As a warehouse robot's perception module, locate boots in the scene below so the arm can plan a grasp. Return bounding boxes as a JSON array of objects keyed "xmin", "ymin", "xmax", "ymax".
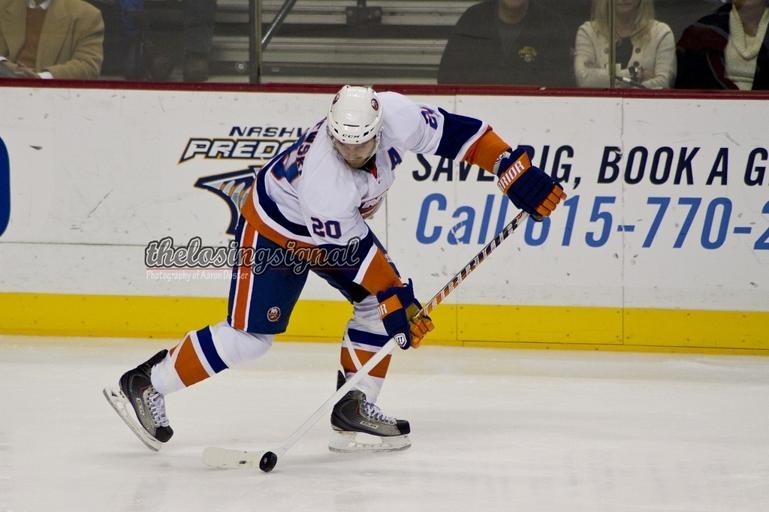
[
  {"xmin": 330, "ymin": 370, "xmax": 411, "ymax": 438},
  {"xmin": 119, "ymin": 348, "xmax": 173, "ymax": 442}
]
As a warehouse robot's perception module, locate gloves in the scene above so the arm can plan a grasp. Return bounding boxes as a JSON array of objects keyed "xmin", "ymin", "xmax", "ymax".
[
  {"xmin": 497, "ymin": 144, "xmax": 567, "ymax": 221},
  {"xmin": 376, "ymin": 278, "xmax": 434, "ymax": 351}
]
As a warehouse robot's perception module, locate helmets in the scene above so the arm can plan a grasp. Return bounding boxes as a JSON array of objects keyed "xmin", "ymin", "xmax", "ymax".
[{"xmin": 326, "ymin": 85, "xmax": 384, "ymax": 167}]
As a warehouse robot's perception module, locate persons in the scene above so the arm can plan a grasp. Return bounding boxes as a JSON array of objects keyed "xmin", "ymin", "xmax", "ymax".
[
  {"xmin": 119, "ymin": 83, "xmax": 570, "ymax": 439},
  {"xmin": 570, "ymin": 0, "xmax": 677, "ymax": 93},
  {"xmin": 437, "ymin": 1, "xmax": 576, "ymax": 90},
  {"xmin": 87, "ymin": 1, "xmax": 219, "ymax": 84},
  {"xmin": 674, "ymin": 0, "xmax": 768, "ymax": 92},
  {"xmin": 0, "ymin": 0, "xmax": 105, "ymax": 83}
]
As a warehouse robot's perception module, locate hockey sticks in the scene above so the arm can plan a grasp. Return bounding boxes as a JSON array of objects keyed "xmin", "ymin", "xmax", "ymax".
[{"xmin": 200, "ymin": 174, "xmax": 571, "ymax": 472}]
